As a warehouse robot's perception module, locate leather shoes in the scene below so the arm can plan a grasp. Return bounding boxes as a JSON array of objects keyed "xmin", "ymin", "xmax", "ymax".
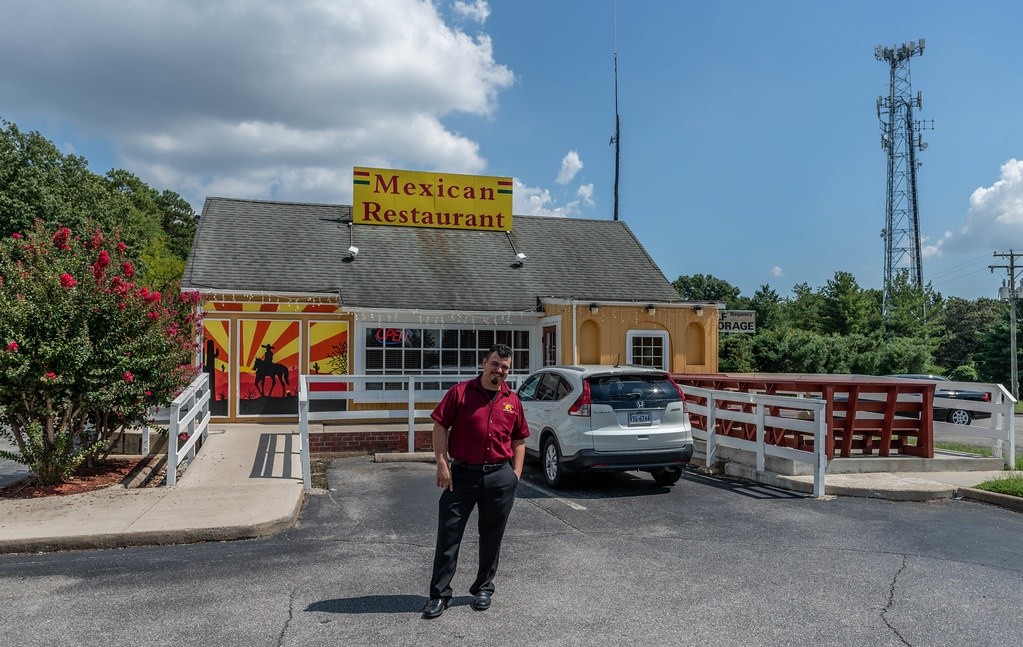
[
  {"xmin": 474, "ymin": 592, "xmax": 491, "ymax": 608},
  {"xmin": 424, "ymin": 597, "xmax": 452, "ymax": 616}
]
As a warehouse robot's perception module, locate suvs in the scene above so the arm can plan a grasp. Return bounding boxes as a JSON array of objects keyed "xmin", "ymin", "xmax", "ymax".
[{"xmin": 514, "ymin": 364, "xmax": 694, "ymax": 486}]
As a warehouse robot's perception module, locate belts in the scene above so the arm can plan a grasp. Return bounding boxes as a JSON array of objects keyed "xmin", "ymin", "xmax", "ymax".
[{"xmin": 452, "ymin": 458, "xmax": 506, "ymax": 472}]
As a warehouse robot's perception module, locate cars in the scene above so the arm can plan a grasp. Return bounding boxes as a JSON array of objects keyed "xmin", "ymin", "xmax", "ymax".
[{"xmin": 813, "ymin": 374, "xmax": 992, "ymax": 425}]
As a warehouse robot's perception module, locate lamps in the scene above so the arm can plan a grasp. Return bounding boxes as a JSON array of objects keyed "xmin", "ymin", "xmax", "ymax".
[
  {"xmin": 348, "ymin": 246, "xmax": 359, "ymax": 258},
  {"xmin": 589, "ymin": 304, "xmax": 598, "ymax": 315},
  {"xmin": 646, "ymin": 306, "xmax": 655, "ymax": 315},
  {"xmin": 515, "ymin": 252, "xmax": 527, "ymax": 266},
  {"xmin": 694, "ymin": 307, "xmax": 703, "ymax": 316}
]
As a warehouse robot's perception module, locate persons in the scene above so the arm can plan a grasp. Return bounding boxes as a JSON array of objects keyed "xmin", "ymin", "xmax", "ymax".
[{"xmin": 424, "ymin": 344, "xmax": 531, "ymax": 619}]
